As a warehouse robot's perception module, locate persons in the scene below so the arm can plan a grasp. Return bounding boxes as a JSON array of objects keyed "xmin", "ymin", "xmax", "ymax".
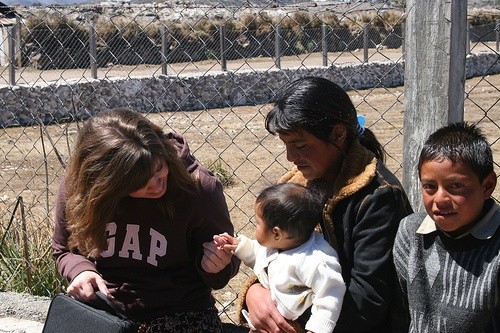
[
  {"xmin": 213, "ymin": 182, "xmax": 348, "ymax": 333},
  {"xmin": 234, "ymin": 76, "xmax": 416, "ymax": 333},
  {"xmin": 49, "ymin": 107, "xmax": 243, "ymax": 333},
  {"xmin": 391, "ymin": 122, "xmax": 500, "ymax": 333}
]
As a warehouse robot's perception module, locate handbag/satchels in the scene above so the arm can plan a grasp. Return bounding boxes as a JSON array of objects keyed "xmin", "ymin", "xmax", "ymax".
[{"xmin": 42, "ymin": 289, "xmax": 139, "ymax": 333}]
